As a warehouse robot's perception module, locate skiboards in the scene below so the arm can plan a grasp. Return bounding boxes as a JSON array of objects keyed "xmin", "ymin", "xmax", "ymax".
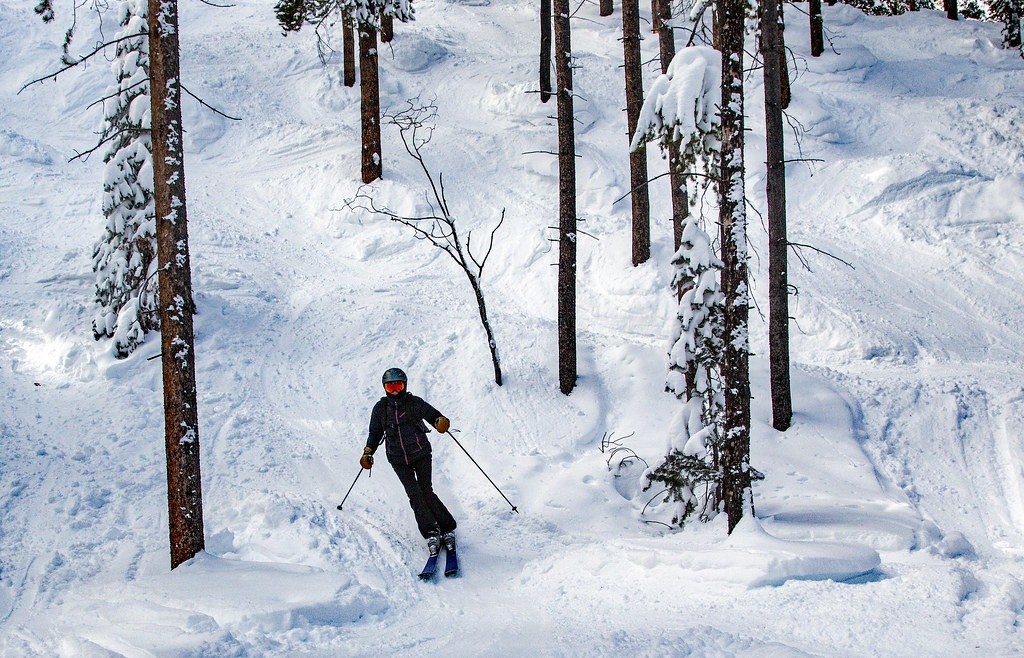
[{"xmin": 417, "ymin": 529, "xmax": 459, "ymax": 580}]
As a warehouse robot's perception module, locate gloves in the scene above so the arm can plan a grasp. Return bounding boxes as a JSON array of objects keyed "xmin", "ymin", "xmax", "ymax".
[
  {"xmin": 435, "ymin": 416, "xmax": 449, "ymax": 433},
  {"xmin": 360, "ymin": 447, "xmax": 373, "ymax": 469}
]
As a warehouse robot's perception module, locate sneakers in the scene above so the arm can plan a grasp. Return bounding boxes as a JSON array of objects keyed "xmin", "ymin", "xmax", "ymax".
[
  {"xmin": 443, "ymin": 531, "xmax": 455, "ymax": 551},
  {"xmin": 428, "ymin": 535, "xmax": 441, "ymax": 554}
]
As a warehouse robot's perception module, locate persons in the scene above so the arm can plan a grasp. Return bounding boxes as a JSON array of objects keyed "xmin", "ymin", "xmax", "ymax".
[{"xmin": 360, "ymin": 368, "xmax": 457, "ymax": 557}]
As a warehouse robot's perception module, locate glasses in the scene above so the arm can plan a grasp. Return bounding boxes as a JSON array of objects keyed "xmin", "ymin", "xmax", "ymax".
[{"xmin": 385, "ymin": 381, "xmax": 404, "ymax": 393}]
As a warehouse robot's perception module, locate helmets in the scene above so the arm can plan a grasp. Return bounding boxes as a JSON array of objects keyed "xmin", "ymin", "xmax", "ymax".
[{"xmin": 382, "ymin": 368, "xmax": 407, "ymax": 385}]
